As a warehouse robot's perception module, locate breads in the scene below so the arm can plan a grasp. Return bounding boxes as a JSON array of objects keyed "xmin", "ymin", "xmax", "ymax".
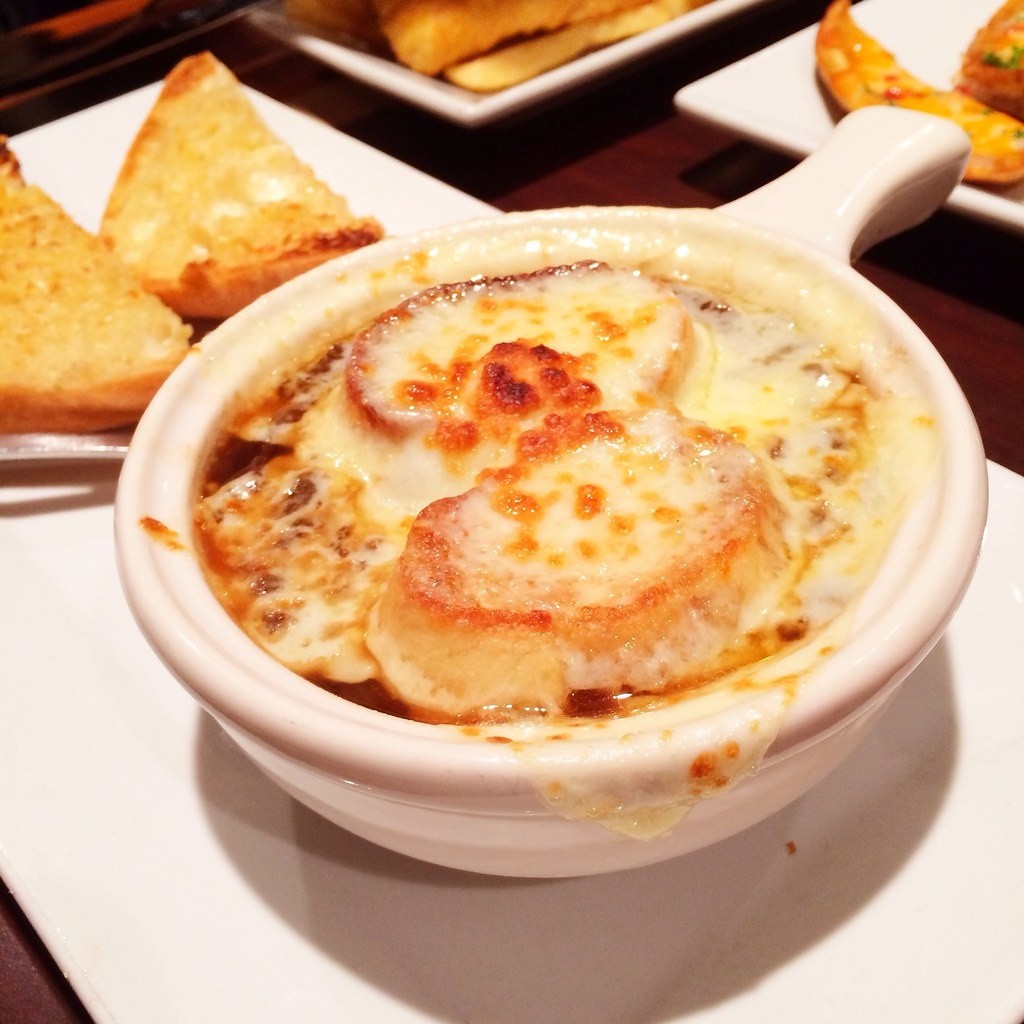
[
  {"xmin": 0, "ymin": 135, "xmax": 195, "ymax": 435},
  {"xmin": 271, "ymin": 1, "xmax": 710, "ymax": 97},
  {"xmin": 92, "ymin": 49, "xmax": 382, "ymax": 324}
]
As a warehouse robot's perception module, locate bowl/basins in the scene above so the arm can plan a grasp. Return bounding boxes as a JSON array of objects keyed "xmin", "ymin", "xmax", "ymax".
[{"xmin": 115, "ymin": 106, "xmax": 990, "ymax": 877}]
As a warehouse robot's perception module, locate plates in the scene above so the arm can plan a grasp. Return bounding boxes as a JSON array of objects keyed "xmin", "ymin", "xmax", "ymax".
[
  {"xmin": 675, "ymin": 0, "xmax": 1024, "ymax": 234},
  {"xmin": 0, "ymin": 72, "xmax": 1024, "ymax": 1024},
  {"xmin": 242, "ymin": 1, "xmax": 764, "ymax": 125}
]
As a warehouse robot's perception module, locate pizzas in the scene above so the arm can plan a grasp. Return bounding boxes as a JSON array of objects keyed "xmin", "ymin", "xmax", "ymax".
[
  {"xmin": 954, "ymin": 0, "xmax": 1024, "ymax": 126},
  {"xmin": 814, "ymin": 0, "xmax": 1024, "ymax": 188}
]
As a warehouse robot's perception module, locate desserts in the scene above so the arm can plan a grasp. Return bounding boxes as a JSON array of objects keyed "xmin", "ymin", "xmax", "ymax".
[{"xmin": 192, "ymin": 248, "xmax": 904, "ymax": 731}]
